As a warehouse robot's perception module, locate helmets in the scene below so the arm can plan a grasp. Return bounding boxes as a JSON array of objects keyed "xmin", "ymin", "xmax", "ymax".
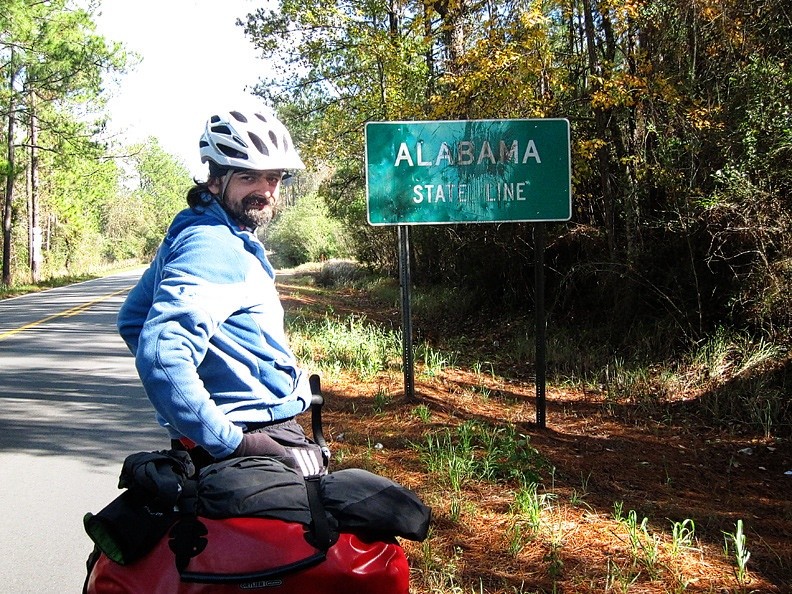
[{"xmin": 198, "ymin": 109, "xmax": 307, "ymax": 171}]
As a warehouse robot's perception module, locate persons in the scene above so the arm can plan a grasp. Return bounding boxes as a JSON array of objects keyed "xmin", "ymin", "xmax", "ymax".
[{"xmin": 116, "ymin": 108, "xmax": 330, "ymax": 477}]
